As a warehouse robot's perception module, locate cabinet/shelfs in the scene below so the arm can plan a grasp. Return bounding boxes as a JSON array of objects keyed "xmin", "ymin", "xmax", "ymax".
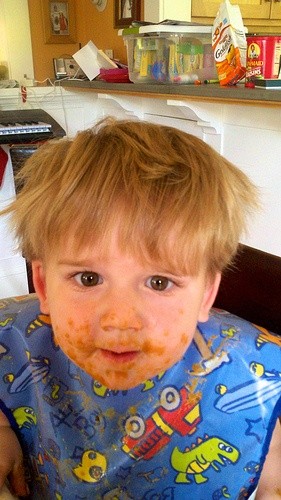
[
  {"xmin": 0, "ymin": 78, "xmax": 281, "ymax": 258},
  {"xmin": 192, "ymin": 0, "xmax": 281, "ymax": 34}
]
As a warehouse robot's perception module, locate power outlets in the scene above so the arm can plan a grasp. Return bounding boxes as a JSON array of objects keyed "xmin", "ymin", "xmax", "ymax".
[{"xmin": 105, "ymin": 50, "xmax": 113, "ymax": 58}]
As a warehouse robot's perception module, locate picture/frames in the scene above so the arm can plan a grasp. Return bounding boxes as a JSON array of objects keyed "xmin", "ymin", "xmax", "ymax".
[
  {"xmin": 40, "ymin": 0, "xmax": 77, "ymax": 44},
  {"xmin": 114, "ymin": 0, "xmax": 144, "ymax": 30}
]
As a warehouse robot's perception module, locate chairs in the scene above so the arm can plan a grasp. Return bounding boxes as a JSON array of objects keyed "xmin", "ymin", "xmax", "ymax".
[{"xmin": 212, "ymin": 243, "xmax": 281, "ymax": 336}]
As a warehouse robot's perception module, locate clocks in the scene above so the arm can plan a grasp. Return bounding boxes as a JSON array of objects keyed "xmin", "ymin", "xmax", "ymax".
[{"xmin": 94, "ymin": 0, "xmax": 107, "ymax": 12}]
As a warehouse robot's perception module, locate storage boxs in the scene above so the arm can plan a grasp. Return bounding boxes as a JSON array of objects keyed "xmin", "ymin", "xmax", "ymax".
[{"xmin": 117, "ymin": 24, "xmax": 248, "ymax": 85}]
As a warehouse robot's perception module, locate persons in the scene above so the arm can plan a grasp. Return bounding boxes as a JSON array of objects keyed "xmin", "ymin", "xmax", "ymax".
[
  {"xmin": 58, "ymin": 11, "xmax": 68, "ymax": 34},
  {"xmin": 0, "ymin": 117, "xmax": 280, "ymax": 500}
]
